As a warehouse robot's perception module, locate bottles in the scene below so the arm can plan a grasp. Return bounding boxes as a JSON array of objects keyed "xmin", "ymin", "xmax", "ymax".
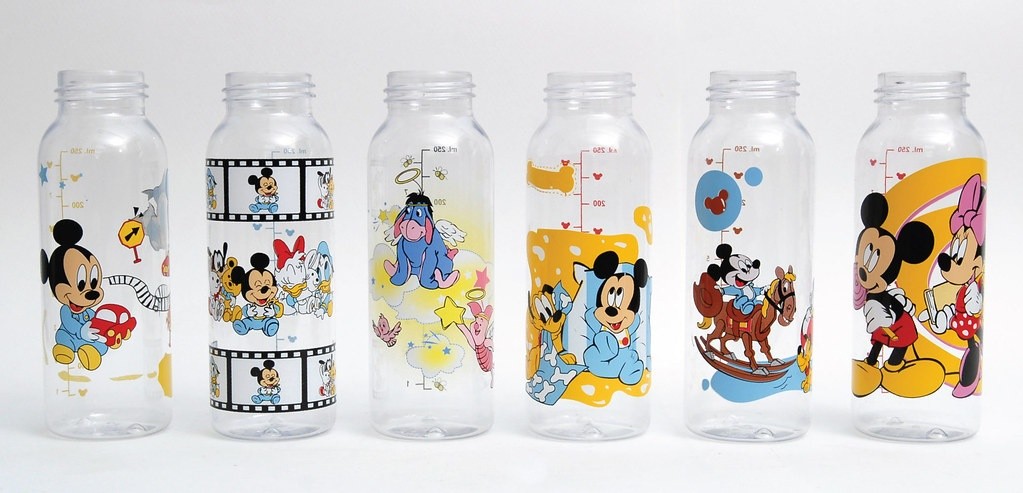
[
  {"xmin": 37, "ymin": 66, "xmax": 171, "ymax": 443},
  {"xmin": 527, "ymin": 70, "xmax": 655, "ymax": 442},
  {"xmin": 203, "ymin": 68, "xmax": 337, "ymax": 439},
  {"xmin": 684, "ymin": 67, "xmax": 816, "ymax": 444},
  {"xmin": 847, "ymin": 68, "xmax": 988, "ymax": 442},
  {"xmin": 364, "ymin": 68, "xmax": 493, "ymax": 445}
]
